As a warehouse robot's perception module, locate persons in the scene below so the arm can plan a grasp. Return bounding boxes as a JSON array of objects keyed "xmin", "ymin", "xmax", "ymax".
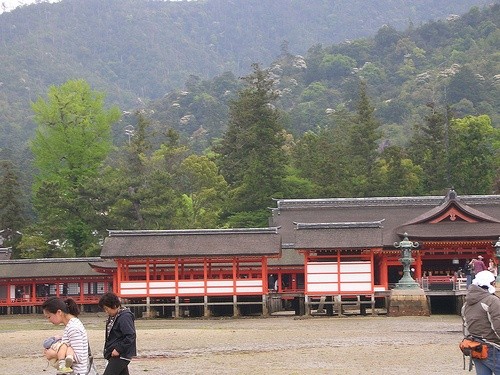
[
  {"xmin": 465, "ymin": 256, "xmax": 498, "ymax": 288},
  {"xmin": 43, "ymin": 337, "xmax": 74, "ymax": 372},
  {"xmin": 459, "ymin": 270, "xmax": 500, "ymax": 375},
  {"xmin": 42, "ymin": 297, "xmax": 93, "ymax": 375},
  {"xmin": 99, "ymin": 293, "xmax": 136, "ymax": 375},
  {"xmin": 268, "ymin": 274, "xmax": 285, "ymax": 291}
]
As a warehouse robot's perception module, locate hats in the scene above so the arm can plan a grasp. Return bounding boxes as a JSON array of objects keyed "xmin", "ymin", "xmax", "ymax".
[
  {"xmin": 471, "ymin": 270, "xmax": 495, "ymax": 294},
  {"xmin": 478, "ymin": 256, "xmax": 483, "ymax": 259}
]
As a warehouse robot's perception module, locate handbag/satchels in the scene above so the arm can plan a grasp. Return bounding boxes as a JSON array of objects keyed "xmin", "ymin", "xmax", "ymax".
[{"xmin": 458, "ymin": 334, "xmax": 488, "ymax": 360}]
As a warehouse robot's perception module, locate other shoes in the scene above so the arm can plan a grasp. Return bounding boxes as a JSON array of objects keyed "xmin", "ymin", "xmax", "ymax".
[{"xmin": 58, "ymin": 355, "xmax": 74, "ymax": 373}]
